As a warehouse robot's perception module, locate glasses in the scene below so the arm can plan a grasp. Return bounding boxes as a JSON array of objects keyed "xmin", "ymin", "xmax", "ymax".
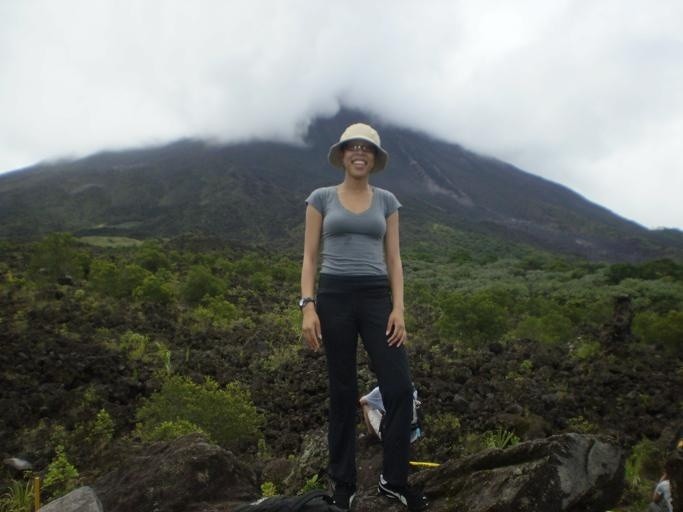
[{"xmin": 343, "ymin": 144, "xmax": 377, "ymax": 154}]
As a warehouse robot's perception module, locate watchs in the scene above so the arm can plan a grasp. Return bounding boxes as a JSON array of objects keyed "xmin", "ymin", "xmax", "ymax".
[{"xmin": 298, "ymin": 297, "xmax": 316, "ymax": 310}]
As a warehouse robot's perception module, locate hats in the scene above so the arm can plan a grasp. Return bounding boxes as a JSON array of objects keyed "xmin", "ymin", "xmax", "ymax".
[{"xmin": 327, "ymin": 123, "xmax": 389, "ymax": 172}]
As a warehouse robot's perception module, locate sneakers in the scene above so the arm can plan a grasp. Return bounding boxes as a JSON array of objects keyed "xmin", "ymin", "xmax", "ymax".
[
  {"xmin": 329, "ymin": 479, "xmax": 359, "ymax": 511},
  {"xmin": 376, "ymin": 473, "xmax": 429, "ymax": 512}
]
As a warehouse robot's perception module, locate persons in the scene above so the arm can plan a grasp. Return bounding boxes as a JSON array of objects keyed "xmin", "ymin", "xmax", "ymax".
[
  {"xmin": 301, "ymin": 122, "xmax": 430, "ymax": 512},
  {"xmin": 648, "ymin": 457, "xmax": 683, "ymax": 512},
  {"xmin": 358, "ymin": 381, "xmax": 422, "ymax": 445}
]
